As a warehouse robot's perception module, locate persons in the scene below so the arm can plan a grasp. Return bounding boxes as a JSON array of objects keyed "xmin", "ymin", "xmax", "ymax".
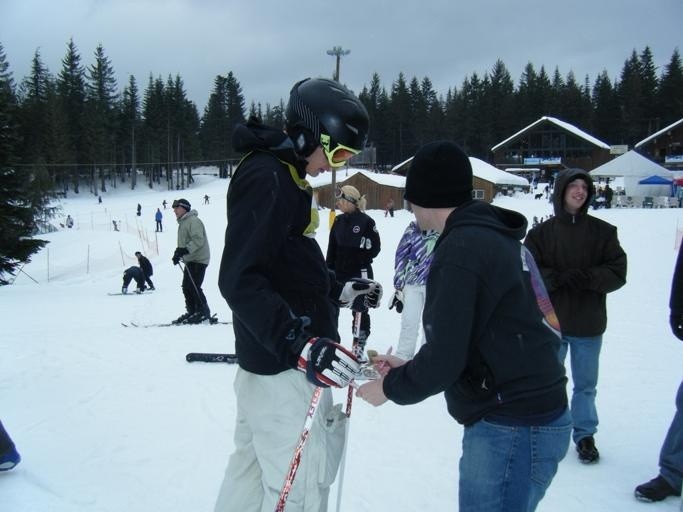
[
  {"xmin": 134, "ymin": 251, "xmax": 155, "ymax": 293},
  {"xmin": 351, "ymin": 138, "xmax": 579, "ymax": 511},
  {"xmin": 543, "ymin": 183, "xmax": 549, "ymax": 200},
  {"xmin": 65, "ymin": 215, "xmax": 72, "ymax": 228},
  {"xmin": 161, "ymin": 199, "xmax": 168, "ymax": 209},
  {"xmin": 212, "ymin": 74, "xmax": 383, "ymax": 512},
  {"xmin": 154, "ymin": 207, "xmax": 163, "ymax": 233},
  {"xmin": 534, "ymin": 191, "xmax": 542, "ymax": 199},
  {"xmin": 135, "ymin": 203, "xmax": 142, "ymax": 216},
  {"xmin": 603, "ymin": 184, "xmax": 613, "ymax": 208},
  {"xmin": 386, "ymin": 196, "xmax": 394, "ymax": 218},
  {"xmin": 525, "ymin": 168, "xmax": 629, "ymax": 466},
  {"xmin": 120, "ymin": 266, "xmax": 144, "ymax": 295},
  {"xmin": 170, "ymin": 198, "xmax": 211, "ymax": 325},
  {"xmin": 632, "ymin": 234, "xmax": 682, "ymax": 503},
  {"xmin": 203, "ymin": 195, "xmax": 210, "ymax": 204},
  {"xmin": 110, "ymin": 219, "xmax": 120, "ymax": 231},
  {"xmin": 386, "ymin": 218, "xmax": 439, "ymax": 364},
  {"xmin": 326, "ymin": 185, "xmax": 380, "ymax": 364},
  {"xmin": 0, "ymin": 419, "xmax": 20, "ymax": 472}
]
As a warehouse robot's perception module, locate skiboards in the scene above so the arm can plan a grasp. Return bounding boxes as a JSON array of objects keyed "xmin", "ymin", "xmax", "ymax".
[
  {"xmin": 109, "ymin": 292, "xmax": 154, "ymax": 296},
  {"xmin": 120, "ymin": 322, "xmax": 235, "ymax": 328}
]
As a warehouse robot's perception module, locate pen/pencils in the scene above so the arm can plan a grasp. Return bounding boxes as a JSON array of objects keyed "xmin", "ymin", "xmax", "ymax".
[{"xmin": 380, "ymin": 345, "xmax": 393, "ymax": 369}]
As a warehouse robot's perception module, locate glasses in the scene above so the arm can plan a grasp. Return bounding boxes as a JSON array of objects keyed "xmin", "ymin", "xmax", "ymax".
[
  {"xmin": 290, "ymin": 77, "xmax": 362, "ymax": 168},
  {"xmin": 172, "ymin": 200, "xmax": 190, "ymax": 208},
  {"xmin": 335, "ymin": 189, "xmax": 357, "ymax": 204}
]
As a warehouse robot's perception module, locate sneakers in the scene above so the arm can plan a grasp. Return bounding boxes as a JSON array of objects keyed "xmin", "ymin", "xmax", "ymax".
[
  {"xmin": 636, "ymin": 476, "xmax": 680, "ymax": 501},
  {"xmin": 577, "ymin": 436, "xmax": 599, "ymax": 462},
  {"xmin": 178, "ymin": 313, "xmax": 210, "ymax": 323}
]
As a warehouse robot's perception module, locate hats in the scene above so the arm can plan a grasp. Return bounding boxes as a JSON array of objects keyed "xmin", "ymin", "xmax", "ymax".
[{"xmin": 402, "ymin": 141, "xmax": 472, "ymax": 208}]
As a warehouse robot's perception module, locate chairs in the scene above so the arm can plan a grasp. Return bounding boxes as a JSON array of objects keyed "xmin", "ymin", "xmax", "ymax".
[{"xmin": 612, "ymin": 195, "xmax": 679, "ymax": 210}]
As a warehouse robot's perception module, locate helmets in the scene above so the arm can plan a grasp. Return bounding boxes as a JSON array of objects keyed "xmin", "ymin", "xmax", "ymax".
[
  {"xmin": 286, "ymin": 79, "xmax": 370, "ymax": 157},
  {"xmin": 178, "ymin": 199, "xmax": 190, "ymax": 211}
]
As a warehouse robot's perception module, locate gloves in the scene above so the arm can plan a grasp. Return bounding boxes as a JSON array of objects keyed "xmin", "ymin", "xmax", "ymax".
[
  {"xmin": 339, "ymin": 277, "xmax": 382, "ymax": 312},
  {"xmin": 172, "ymin": 248, "xmax": 188, "ymax": 265},
  {"xmin": 297, "ymin": 336, "xmax": 359, "ymax": 388},
  {"xmin": 388, "ymin": 289, "xmax": 404, "ymax": 313},
  {"xmin": 557, "ymin": 269, "xmax": 592, "ymax": 292}
]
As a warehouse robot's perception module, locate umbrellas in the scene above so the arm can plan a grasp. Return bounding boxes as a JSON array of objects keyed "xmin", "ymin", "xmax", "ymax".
[{"xmin": 637, "ymin": 173, "xmax": 673, "ymax": 207}]
